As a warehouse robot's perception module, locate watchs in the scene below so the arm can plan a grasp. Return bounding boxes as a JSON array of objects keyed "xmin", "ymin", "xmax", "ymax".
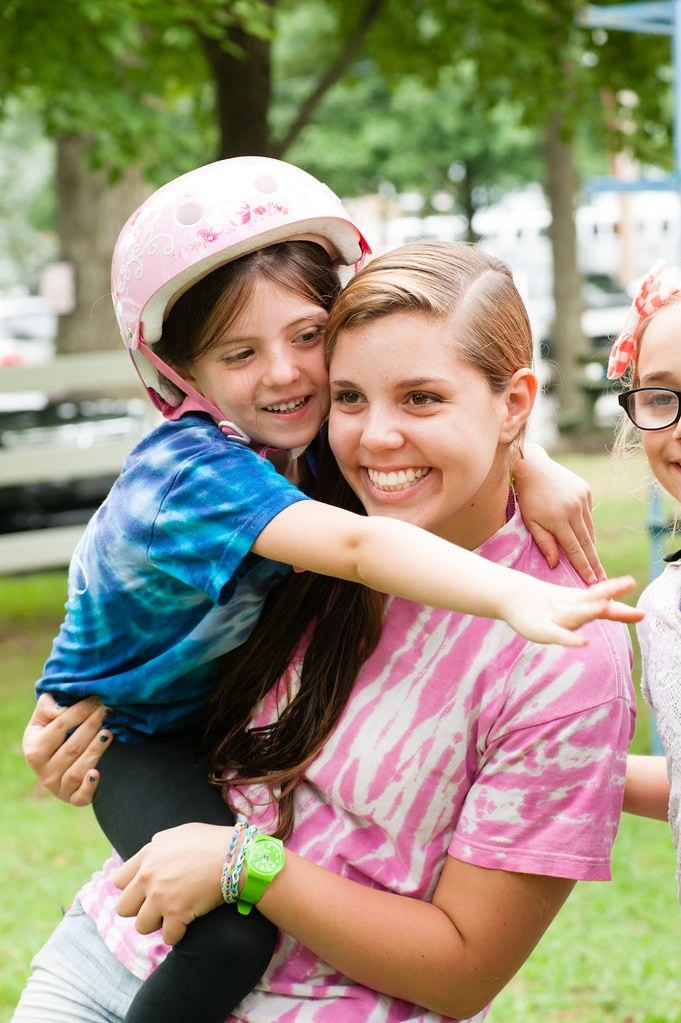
[{"xmin": 236, "ymin": 832, "xmax": 286, "ymax": 916}]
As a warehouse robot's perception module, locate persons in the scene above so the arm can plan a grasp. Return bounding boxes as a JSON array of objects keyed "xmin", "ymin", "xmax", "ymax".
[
  {"xmin": 42, "ymin": 149, "xmax": 648, "ymax": 1019},
  {"xmin": 6, "ymin": 235, "xmax": 633, "ymax": 1023},
  {"xmin": 599, "ymin": 250, "xmax": 681, "ymax": 943}
]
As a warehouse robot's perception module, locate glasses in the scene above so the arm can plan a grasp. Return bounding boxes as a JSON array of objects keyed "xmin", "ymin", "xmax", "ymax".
[{"xmin": 618, "ymin": 386, "xmax": 680, "ymax": 431}]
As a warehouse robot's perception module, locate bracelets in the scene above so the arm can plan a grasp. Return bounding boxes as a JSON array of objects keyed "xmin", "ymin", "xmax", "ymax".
[
  {"xmin": 221, "ymin": 818, "xmax": 250, "ymax": 905},
  {"xmin": 230, "ymin": 822, "xmax": 259, "ymax": 898}
]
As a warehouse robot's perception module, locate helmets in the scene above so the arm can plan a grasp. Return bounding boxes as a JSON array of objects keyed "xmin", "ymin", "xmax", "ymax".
[{"xmin": 111, "ymin": 155, "xmax": 372, "ymax": 407}]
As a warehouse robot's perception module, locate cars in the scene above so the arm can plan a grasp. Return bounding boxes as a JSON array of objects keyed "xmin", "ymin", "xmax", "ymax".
[{"xmin": 1, "ymin": 195, "xmax": 647, "ymax": 533}]
